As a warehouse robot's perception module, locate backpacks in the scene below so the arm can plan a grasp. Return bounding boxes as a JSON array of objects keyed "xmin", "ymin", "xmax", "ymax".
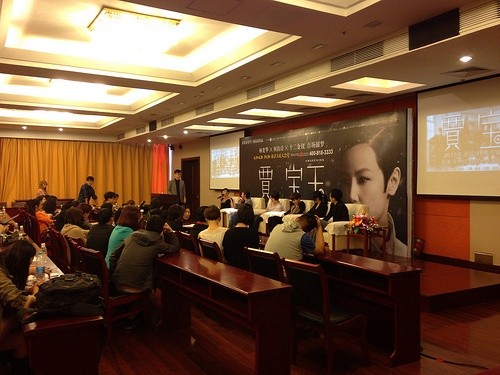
[{"xmin": 36, "ymin": 272, "xmax": 104, "ymax": 318}]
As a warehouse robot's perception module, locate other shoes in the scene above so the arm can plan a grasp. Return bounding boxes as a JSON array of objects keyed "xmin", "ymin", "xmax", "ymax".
[{"xmin": 124, "ymin": 319, "xmax": 136, "ymax": 329}]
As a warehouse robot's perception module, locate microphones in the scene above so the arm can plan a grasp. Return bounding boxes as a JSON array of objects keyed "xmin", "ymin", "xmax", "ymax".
[{"xmin": 217, "ymin": 194, "xmax": 224, "ymax": 199}]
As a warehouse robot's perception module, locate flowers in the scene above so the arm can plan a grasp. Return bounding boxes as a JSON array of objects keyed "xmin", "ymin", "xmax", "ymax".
[{"xmin": 345, "ymin": 213, "xmax": 378, "ymax": 235}]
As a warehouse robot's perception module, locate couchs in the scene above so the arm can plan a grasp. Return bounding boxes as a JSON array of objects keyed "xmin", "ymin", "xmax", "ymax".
[{"xmin": 219, "ymin": 197, "xmax": 371, "ymax": 250}]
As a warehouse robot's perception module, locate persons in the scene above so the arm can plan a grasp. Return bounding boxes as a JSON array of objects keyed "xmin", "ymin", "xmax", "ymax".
[
  {"xmin": 338, "ymin": 134, "xmax": 409, "ymax": 259},
  {"xmin": 0, "ymin": 170, "xmax": 350, "ymax": 375}
]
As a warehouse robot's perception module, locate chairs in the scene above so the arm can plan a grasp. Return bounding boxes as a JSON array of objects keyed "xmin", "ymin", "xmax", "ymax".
[
  {"xmin": 283, "ymin": 258, "xmax": 369, "ymax": 375},
  {"xmin": 18, "ymin": 208, "xmax": 220, "ymax": 341},
  {"xmin": 244, "ymin": 246, "xmax": 286, "ymax": 284}
]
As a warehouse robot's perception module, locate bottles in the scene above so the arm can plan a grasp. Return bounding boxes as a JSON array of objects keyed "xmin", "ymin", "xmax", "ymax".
[
  {"xmin": 36, "ymin": 252, "xmax": 44, "ymax": 275},
  {"xmin": 41, "ymin": 243, "xmax": 47, "ymax": 264},
  {"xmin": 18, "ymin": 226, "xmax": 25, "ymax": 239},
  {"xmin": 13, "ymin": 223, "xmax": 18, "ymax": 232}
]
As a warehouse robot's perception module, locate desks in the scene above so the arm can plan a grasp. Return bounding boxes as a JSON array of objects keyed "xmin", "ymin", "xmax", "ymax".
[
  {"xmin": 344, "ymin": 225, "xmax": 390, "ymax": 261},
  {"xmin": 0, "ymin": 213, "xmax": 104, "ymax": 375},
  {"xmin": 154, "ymin": 248, "xmax": 293, "ymax": 375},
  {"xmin": 259, "ymin": 234, "xmax": 422, "ymax": 369}
]
